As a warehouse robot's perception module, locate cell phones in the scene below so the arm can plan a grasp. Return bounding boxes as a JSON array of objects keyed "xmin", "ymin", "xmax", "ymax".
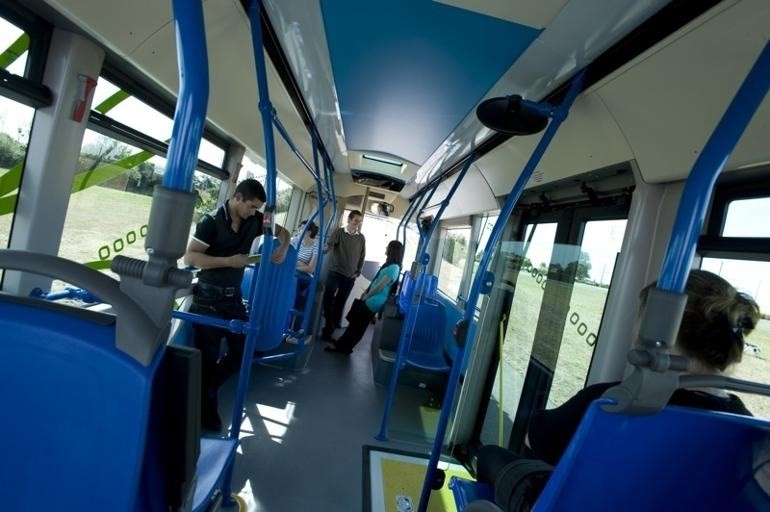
[{"xmin": 248, "ymin": 254, "xmax": 262, "ymax": 263}]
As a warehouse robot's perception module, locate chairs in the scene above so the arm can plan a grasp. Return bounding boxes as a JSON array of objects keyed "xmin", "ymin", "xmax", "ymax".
[
  {"xmin": 195, "ymin": 235, "xmax": 316, "ymax": 372},
  {"xmin": 378, "ymin": 268, "xmax": 452, "ymax": 385},
  {"xmin": 1, "ymin": 247, "xmax": 238, "ymax": 511},
  {"xmin": 446, "ymin": 373, "xmax": 769, "ymax": 510}
]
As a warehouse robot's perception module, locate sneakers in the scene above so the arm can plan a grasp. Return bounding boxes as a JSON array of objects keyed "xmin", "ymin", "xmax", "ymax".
[
  {"xmin": 199, "ymin": 368, "xmax": 226, "ymax": 431},
  {"xmin": 465, "ymin": 437, "xmax": 484, "ymax": 474},
  {"xmin": 286, "ymin": 332, "xmax": 353, "ymax": 352}
]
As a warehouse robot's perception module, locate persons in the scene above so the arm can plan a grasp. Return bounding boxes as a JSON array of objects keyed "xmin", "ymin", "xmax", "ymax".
[
  {"xmin": 178, "ymin": 178, "xmax": 292, "ymax": 432},
  {"xmin": 319, "ymin": 209, "xmax": 366, "ymax": 343},
  {"xmin": 475, "ymin": 266, "xmax": 761, "ymax": 512},
  {"xmin": 289, "ymin": 218, "xmax": 319, "ymax": 310},
  {"xmin": 322, "ymin": 239, "xmax": 404, "ymax": 355}
]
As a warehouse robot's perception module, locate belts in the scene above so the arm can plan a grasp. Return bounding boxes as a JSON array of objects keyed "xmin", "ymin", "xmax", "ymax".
[{"xmin": 197, "ymin": 279, "xmax": 242, "ymax": 297}]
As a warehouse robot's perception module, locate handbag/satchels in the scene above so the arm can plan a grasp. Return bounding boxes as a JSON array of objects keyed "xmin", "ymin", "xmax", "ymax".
[{"xmin": 347, "ymin": 298, "xmax": 373, "ymax": 329}]
